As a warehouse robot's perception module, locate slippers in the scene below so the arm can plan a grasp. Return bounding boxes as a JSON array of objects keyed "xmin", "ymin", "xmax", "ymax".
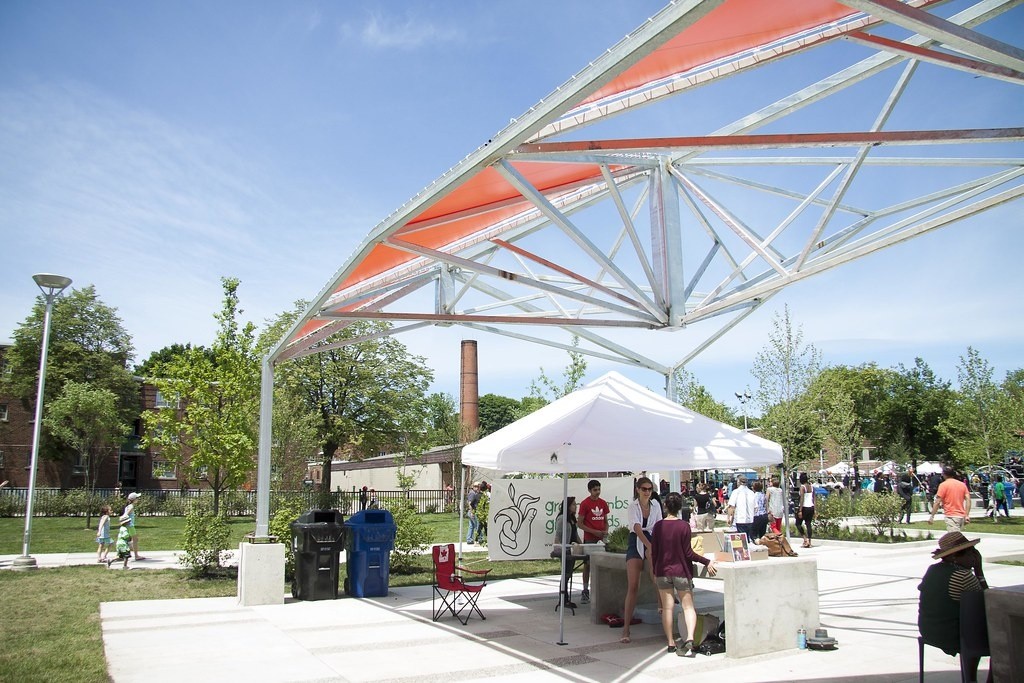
[
  {"xmin": 621, "ymin": 632, "xmax": 631, "ymax": 643},
  {"xmin": 658, "ymin": 607, "xmax": 663, "ymax": 614}
]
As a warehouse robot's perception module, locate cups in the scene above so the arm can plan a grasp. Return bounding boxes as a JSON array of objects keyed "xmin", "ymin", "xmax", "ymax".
[{"xmin": 797, "ymin": 629, "xmax": 807, "ymax": 649}]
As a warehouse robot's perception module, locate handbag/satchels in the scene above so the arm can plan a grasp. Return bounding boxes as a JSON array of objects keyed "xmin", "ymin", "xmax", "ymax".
[
  {"xmin": 677, "ymin": 611, "xmax": 725, "ymax": 656},
  {"xmin": 759, "ymin": 533, "xmax": 797, "ymax": 556},
  {"xmin": 708, "ymin": 493, "xmax": 717, "ymax": 513}
]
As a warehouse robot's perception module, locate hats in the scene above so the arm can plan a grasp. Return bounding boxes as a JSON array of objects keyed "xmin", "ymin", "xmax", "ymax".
[
  {"xmin": 119, "ymin": 515, "xmax": 131, "ymax": 525},
  {"xmin": 931, "ymin": 532, "xmax": 981, "ymax": 560},
  {"xmin": 128, "ymin": 492, "xmax": 141, "ymax": 501},
  {"xmin": 737, "ymin": 475, "xmax": 748, "ymax": 483}
]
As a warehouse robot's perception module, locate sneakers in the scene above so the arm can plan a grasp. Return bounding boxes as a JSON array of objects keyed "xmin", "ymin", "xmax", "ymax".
[{"xmin": 581, "ymin": 590, "xmax": 589, "ymax": 604}]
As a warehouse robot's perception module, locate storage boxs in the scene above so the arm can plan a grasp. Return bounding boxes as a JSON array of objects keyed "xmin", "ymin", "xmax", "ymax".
[
  {"xmin": 636, "ymin": 603, "xmax": 680, "ymax": 624},
  {"xmin": 581, "ymin": 543, "xmax": 606, "ymax": 556},
  {"xmin": 750, "ymin": 545, "xmax": 768, "ymax": 561}
]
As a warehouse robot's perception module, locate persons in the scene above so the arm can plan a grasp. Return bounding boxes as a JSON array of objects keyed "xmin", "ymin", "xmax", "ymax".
[
  {"xmin": 765, "ymin": 477, "xmax": 783, "ymax": 537},
  {"xmin": 896, "ymin": 475, "xmax": 913, "ymax": 524},
  {"xmin": 577, "ymin": 480, "xmax": 609, "ymax": 603},
  {"xmin": 620, "ymin": 477, "xmax": 663, "ymax": 642},
  {"xmin": 650, "ymin": 493, "xmax": 718, "ymax": 653},
  {"xmin": 445, "ymin": 484, "xmax": 453, "ymax": 504},
  {"xmin": 125, "ymin": 491, "xmax": 146, "ymax": 561},
  {"xmin": 954, "ymin": 467, "xmax": 1024, "ymax": 517},
  {"xmin": 359, "ymin": 486, "xmax": 376, "ymax": 512},
  {"xmin": 794, "ymin": 472, "xmax": 814, "ymax": 551},
  {"xmin": 107, "ymin": 514, "xmax": 133, "ymax": 569},
  {"xmin": 929, "ymin": 466, "xmax": 971, "ymax": 533},
  {"xmin": 727, "ymin": 475, "xmax": 759, "ymax": 545},
  {"xmin": 690, "ymin": 480, "xmax": 726, "ymax": 517},
  {"xmin": 917, "ymin": 532, "xmax": 994, "ymax": 683},
  {"xmin": 814, "ymin": 468, "xmax": 893, "ymax": 496},
  {"xmin": 115, "ymin": 480, "xmax": 125, "ymax": 498},
  {"xmin": 555, "ymin": 496, "xmax": 583, "ymax": 608},
  {"xmin": 95, "ymin": 506, "xmax": 114, "ymax": 564},
  {"xmin": 464, "ymin": 480, "xmax": 491, "ymax": 545},
  {"xmin": 749, "ymin": 481, "xmax": 768, "ymax": 542}
]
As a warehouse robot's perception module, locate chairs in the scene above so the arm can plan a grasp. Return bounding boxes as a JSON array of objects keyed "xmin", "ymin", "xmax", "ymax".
[{"xmin": 432, "ymin": 543, "xmax": 492, "ymax": 626}]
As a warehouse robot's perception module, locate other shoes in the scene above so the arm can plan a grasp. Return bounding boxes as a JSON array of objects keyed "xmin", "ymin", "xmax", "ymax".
[
  {"xmin": 107, "ymin": 559, "xmax": 111, "ymax": 568},
  {"xmin": 135, "ymin": 557, "xmax": 145, "ymax": 561},
  {"xmin": 562, "ymin": 601, "xmax": 576, "ymax": 608},
  {"xmin": 667, "ymin": 645, "xmax": 677, "ymax": 652},
  {"xmin": 97, "ymin": 558, "xmax": 110, "ymax": 563},
  {"xmin": 801, "ymin": 542, "xmax": 812, "ymax": 548},
  {"xmin": 676, "ymin": 640, "xmax": 694, "ymax": 656},
  {"xmin": 123, "ymin": 566, "xmax": 129, "ymax": 570}
]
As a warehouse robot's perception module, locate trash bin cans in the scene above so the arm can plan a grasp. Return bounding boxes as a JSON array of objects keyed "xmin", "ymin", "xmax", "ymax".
[
  {"xmin": 980, "ymin": 483, "xmax": 1015, "ymax": 509},
  {"xmin": 790, "ymin": 486, "xmax": 828, "ymax": 518},
  {"xmin": 289, "ymin": 510, "xmax": 398, "ymax": 601}
]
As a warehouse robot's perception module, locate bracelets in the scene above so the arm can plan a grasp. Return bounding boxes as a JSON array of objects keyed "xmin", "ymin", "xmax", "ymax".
[
  {"xmin": 930, "ymin": 515, "xmax": 934, "ymax": 518},
  {"xmin": 976, "ymin": 575, "xmax": 987, "ymax": 582}
]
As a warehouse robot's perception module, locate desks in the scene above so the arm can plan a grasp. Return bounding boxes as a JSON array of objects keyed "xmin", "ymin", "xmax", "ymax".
[
  {"xmin": 550, "ymin": 551, "xmax": 589, "ymax": 616},
  {"xmin": 590, "ymin": 550, "xmax": 818, "ymax": 659}
]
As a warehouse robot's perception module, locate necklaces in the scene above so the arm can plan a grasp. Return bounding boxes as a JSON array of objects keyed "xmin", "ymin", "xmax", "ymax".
[{"xmin": 639, "ymin": 502, "xmax": 650, "ymax": 510}]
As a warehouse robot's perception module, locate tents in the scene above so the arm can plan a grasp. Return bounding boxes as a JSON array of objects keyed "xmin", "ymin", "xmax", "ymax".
[
  {"xmin": 869, "ymin": 458, "xmax": 914, "ymax": 476},
  {"xmin": 456, "ymin": 367, "xmax": 789, "ymax": 650},
  {"xmin": 915, "ymin": 459, "xmax": 943, "ymax": 475},
  {"xmin": 820, "ymin": 461, "xmax": 866, "ymax": 478}
]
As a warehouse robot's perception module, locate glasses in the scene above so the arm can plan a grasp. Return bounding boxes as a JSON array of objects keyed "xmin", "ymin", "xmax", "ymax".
[{"xmin": 639, "ymin": 487, "xmax": 652, "ymax": 492}]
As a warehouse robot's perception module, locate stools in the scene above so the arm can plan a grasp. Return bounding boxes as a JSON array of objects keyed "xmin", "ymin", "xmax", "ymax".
[{"xmin": 918, "ymin": 635, "xmax": 990, "ymax": 682}]
